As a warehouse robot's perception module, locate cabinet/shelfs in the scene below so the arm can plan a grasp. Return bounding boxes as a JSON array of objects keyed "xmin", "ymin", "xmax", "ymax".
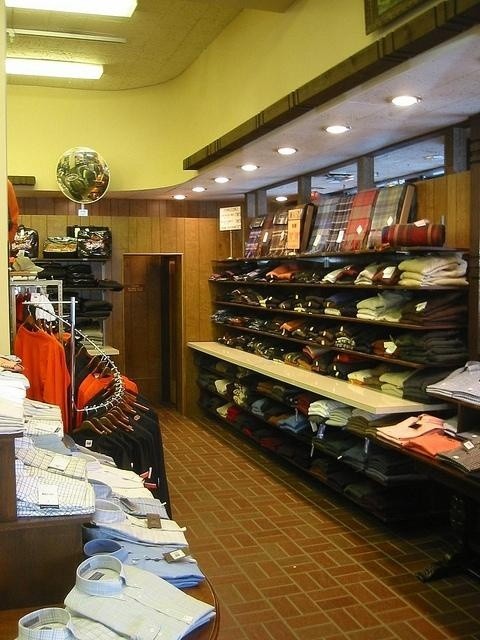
[
  {"xmin": 375, "ymin": 392, "xmax": 479, "ymax": 584},
  {"xmin": 193, "ymin": 248, "xmax": 480, "ymax": 526},
  {"xmin": 30, "ymin": 258, "xmax": 119, "ymax": 356},
  {"xmin": 0, "ymin": 432, "xmax": 220, "ymax": 640}
]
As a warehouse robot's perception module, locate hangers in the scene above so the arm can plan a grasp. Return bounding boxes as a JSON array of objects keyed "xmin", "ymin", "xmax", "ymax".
[{"xmin": 23, "ymin": 303, "xmax": 150, "ymax": 436}]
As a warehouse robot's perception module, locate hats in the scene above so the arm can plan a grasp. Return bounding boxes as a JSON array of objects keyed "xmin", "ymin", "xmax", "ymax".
[{"xmin": 12, "ymin": 257, "xmax": 44, "ymax": 273}]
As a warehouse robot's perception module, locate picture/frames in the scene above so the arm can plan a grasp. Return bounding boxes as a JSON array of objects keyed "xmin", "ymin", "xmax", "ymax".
[{"xmin": 363, "ymin": 0, "xmax": 431, "ymax": 36}]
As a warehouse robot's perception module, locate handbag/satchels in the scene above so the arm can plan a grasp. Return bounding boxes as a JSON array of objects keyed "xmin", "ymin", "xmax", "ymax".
[
  {"xmin": 10, "ymin": 225, "xmax": 38, "ymax": 257},
  {"xmin": 78, "ymin": 230, "xmax": 112, "ymax": 258},
  {"xmin": 43, "ymin": 236, "xmax": 77, "ymax": 258}
]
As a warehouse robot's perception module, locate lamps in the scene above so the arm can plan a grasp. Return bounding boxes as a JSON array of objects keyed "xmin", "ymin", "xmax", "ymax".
[
  {"xmin": 5, "ymin": 58, "xmax": 104, "ymax": 82},
  {"xmin": 4, "ymin": 0, "xmax": 138, "ymax": 18}
]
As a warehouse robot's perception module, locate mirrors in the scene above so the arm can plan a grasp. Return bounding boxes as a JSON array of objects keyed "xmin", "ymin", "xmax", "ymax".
[{"xmin": 56, "ymin": 146, "xmax": 111, "ymax": 204}]
